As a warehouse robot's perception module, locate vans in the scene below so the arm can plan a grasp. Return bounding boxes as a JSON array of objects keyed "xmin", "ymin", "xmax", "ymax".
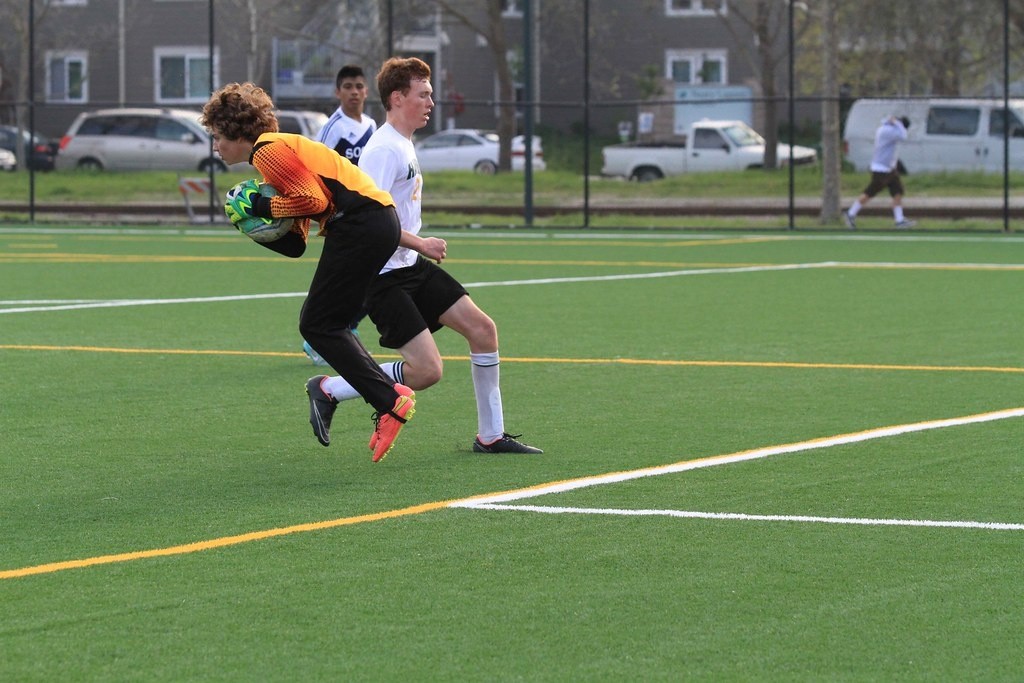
[{"xmin": 843, "ymin": 100, "xmax": 1024, "ymax": 178}]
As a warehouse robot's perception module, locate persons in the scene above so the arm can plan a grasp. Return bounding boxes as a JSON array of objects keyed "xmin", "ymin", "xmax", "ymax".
[
  {"xmin": 304, "ymin": 57, "xmax": 544, "ymax": 454},
  {"xmin": 197, "ymin": 80, "xmax": 417, "ymax": 464},
  {"xmin": 302, "ymin": 65, "xmax": 377, "ymax": 367},
  {"xmin": 842, "ymin": 115, "xmax": 916, "ymax": 230}
]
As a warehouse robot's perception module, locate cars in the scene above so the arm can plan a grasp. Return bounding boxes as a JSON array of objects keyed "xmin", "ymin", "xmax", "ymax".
[
  {"xmin": 413, "ymin": 126, "xmax": 548, "ymax": 176},
  {"xmin": 0, "ymin": 126, "xmax": 51, "ymax": 171},
  {"xmin": 51, "ymin": 105, "xmax": 239, "ymax": 176},
  {"xmin": 272, "ymin": 109, "xmax": 328, "ymax": 148}
]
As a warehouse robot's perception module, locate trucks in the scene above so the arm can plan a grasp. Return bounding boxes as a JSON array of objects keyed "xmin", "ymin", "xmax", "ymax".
[{"xmin": 601, "ymin": 119, "xmax": 819, "ymax": 188}]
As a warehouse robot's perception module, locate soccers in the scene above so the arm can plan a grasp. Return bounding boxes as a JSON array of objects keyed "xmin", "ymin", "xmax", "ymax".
[{"xmin": 236, "ymin": 181, "xmax": 295, "ymax": 243}]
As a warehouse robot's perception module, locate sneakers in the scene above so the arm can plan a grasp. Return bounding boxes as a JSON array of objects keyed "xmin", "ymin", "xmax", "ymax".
[
  {"xmin": 303, "ymin": 340, "xmax": 330, "ymax": 365},
  {"xmin": 368, "ymin": 383, "xmax": 416, "ymax": 450},
  {"xmin": 473, "ymin": 433, "xmax": 543, "ymax": 453},
  {"xmin": 373, "ymin": 395, "xmax": 416, "ymax": 462},
  {"xmin": 305, "ymin": 375, "xmax": 340, "ymax": 446}
]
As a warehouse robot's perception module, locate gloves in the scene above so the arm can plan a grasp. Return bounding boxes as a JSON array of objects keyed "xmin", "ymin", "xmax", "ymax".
[{"xmin": 224, "ymin": 179, "xmax": 261, "ymax": 224}]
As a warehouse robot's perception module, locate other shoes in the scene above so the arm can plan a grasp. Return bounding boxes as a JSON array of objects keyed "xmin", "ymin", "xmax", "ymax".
[
  {"xmin": 897, "ymin": 218, "xmax": 916, "ymax": 231},
  {"xmin": 844, "ymin": 209, "xmax": 857, "ymax": 229}
]
